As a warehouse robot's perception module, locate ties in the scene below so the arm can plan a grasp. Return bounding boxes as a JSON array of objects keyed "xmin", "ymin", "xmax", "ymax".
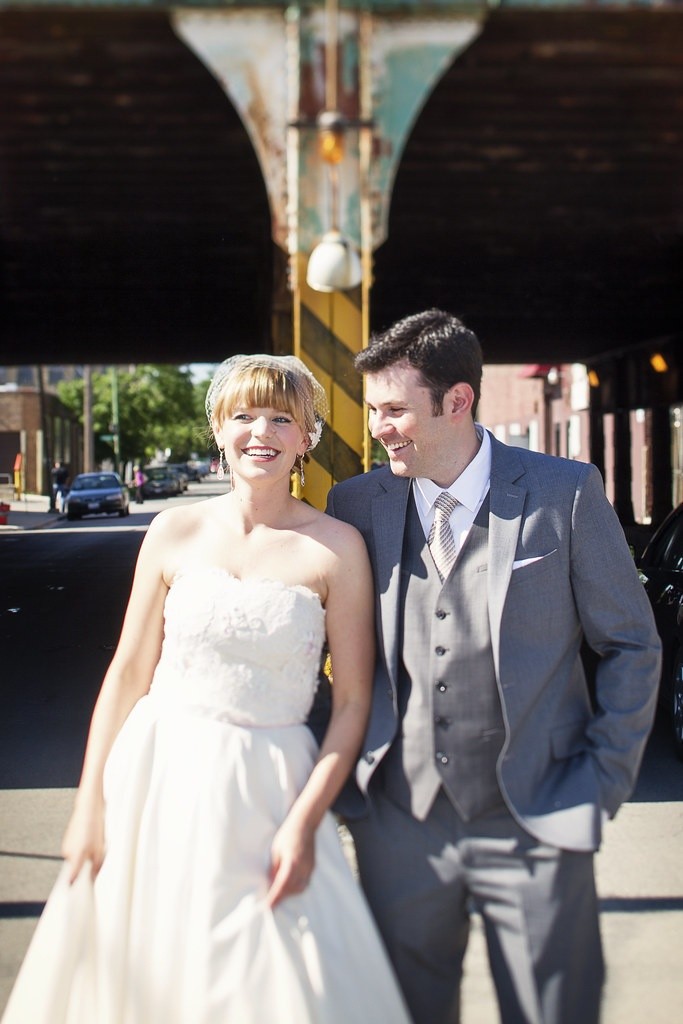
[{"xmin": 425, "ymin": 493, "xmax": 456, "ymax": 578}]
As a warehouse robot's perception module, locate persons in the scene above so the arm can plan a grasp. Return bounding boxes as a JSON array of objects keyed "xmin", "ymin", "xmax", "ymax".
[
  {"xmin": 1, "ymin": 355, "xmax": 418, "ymax": 1024},
  {"xmin": 132, "ymin": 465, "xmax": 145, "ymax": 505},
  {"xmin": 49, "ymin": 460, "xmax": 69, "ymax": 514},
  {"xmin": 323, "ymin": 309, "xmax": 663, "ymax": 1023}
]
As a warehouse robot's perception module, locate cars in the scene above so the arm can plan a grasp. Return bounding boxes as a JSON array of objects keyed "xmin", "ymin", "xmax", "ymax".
[
  {"xmin": 166, "ymin": 459, "xmax": 210, "ymax": 496},
  {"xmin": 68, "ymin": 472, "xmax": 131, "ymax": 522},
  {"xmin": 141, "ymin": 463, "xmax": 182, "ymax": 499},
  {"xmin": 616, "ymin": 499, "xmax": 683, "ymax": 744}
]
